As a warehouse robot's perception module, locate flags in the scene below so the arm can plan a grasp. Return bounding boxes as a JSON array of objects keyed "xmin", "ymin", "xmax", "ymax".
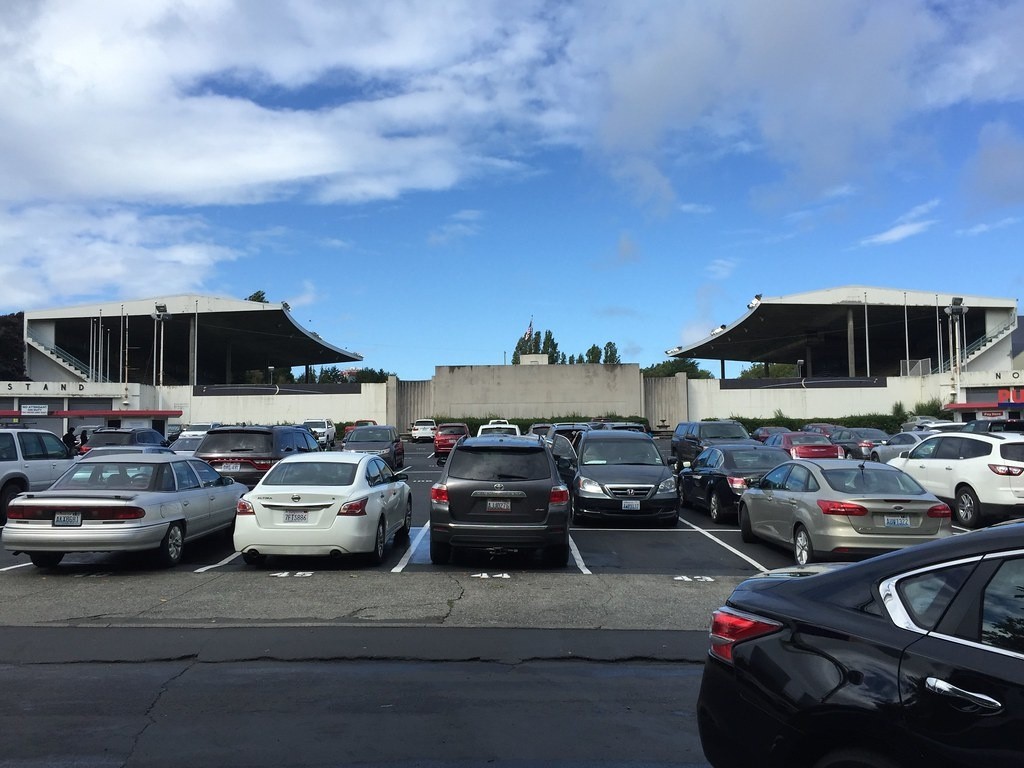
[{"xmin": 526, "ymin": 321, "xmax": 532, "ymax": 340}]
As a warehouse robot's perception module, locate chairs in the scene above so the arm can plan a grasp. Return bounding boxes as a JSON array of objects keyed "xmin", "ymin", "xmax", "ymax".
[
  {"xmin": 107, "ymin": 474, "xmax": 130, "ymax": 487},
  {"xmin": 164, "ymin": 474, "xmax": 187, "ymax": 491},
  {"xmin": 852, "ymin": 473, "xmax": 872, "ymax": 493}
]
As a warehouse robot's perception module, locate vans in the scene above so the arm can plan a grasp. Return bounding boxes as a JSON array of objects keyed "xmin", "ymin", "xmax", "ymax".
[
  {"xmin": 671, "ymin": 421, "xmax": 755, "ymax": 466},
  {"xmin": 431, "ymin": 423, "xmax": 472, "ymax": 458}
]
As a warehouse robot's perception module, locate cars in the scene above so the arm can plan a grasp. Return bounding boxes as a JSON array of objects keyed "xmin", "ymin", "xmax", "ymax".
[
  {"xmin": 343, "ymin": 421, "xmax": 379, "ymax": 442},
  {"xmin": 798, "ymin": 423, "xmax": 847, "ymax": 440},
  {"xmin": 678, "ymin": 444, "xmax": 794, "ymax": 524},
  {"xmin": 0, "ymin": 451, "xmax": 250, "ymax": 570},
  {"xmin": 737, "ymin": 459, "xmax": 954, "ymax": 566},
  {"xmin": 899, "ymin": 414, "xmax": 1024, "ymax": 433},
  {"xmin": 479, "ymin": 418, "xmax": 652, "ymax": 441},
  {"xmin": 696, "ymin": 512, "xmax": 1024, "ymax": 768},
  {"xmin": 77, "ymin": 423, "xmax": 219, "ymax": 467},
  {"xmin": 825, "ymin": 428, "xmax": 893, "ymax": 461},
  {"xmin": 750, "ymin": 427, "xmax": 792, "ymax": 446},
  {"xmin": 410, "ymin": 418, "xmax": 440, "ymax": 443},
  {"xmin": 871, "ymin": 429, "xmax": 942, "ymax": 466},
  {"xmin": 233, "ymin": 452, "xmax": 414, "ymax": 566},
  {"xmin": 764, "ymin": 430, "xmax": 845, "ymax": 461}
]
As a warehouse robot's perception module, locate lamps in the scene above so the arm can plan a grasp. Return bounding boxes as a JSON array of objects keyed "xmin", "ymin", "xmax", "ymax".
[
  {"xmin": 710, "ymin": 324, "xmax": 726, "ymax": 336},
  {"xmin": 747, "ymin": 294, "xmax": 763, "ymax": 309},
  {"xmin": 665, "ymin": 345, "xmax": 682, "ymax": 354},
  {"xmin": 156, "ymin": 303, "xmax": 167, "ymax": 313}
]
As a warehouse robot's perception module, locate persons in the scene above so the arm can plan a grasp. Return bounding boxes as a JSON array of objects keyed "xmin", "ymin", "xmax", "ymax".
[
  {"xmin": 80, "ymin": 430, "xmax": 88, "ymax": 445},
  {"xmin": 63, "ymin": 427, "xmax": 77, "ymax": 450}
]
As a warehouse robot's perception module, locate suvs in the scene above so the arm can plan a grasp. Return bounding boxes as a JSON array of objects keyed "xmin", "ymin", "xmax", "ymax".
[
  {"xmin": 191, "ymin": 424, "xmax": 327, "ymax": 491},
  {"xmin": 304, "ymin": 419, "xmax": 337, "ymax": 450},
  {"xmin": 554, "ymin": 431, "xmax": 680, "ymax": 527},
  {"xmin": 0, "ymin": 427, "xmax": 83, "ymax": 529},
  {"xmin": 430, "ymin": 433, "xmax": 573, "ymax": 563},
  {"xmin": 342, "ymin": 424, "xmax": 405, "ymax": 472},
  {"xmin": 886, "ymin": 430, "xmax": 1024, "ymax": 529}
]
